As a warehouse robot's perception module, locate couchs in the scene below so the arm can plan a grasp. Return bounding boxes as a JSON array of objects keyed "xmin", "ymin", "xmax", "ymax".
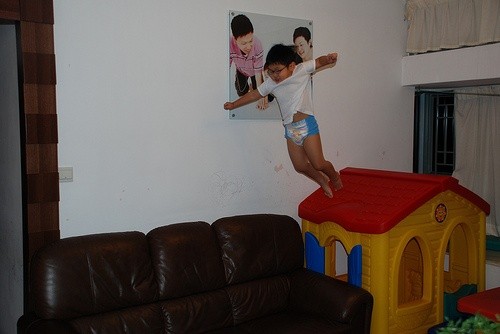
[{"xmin": 17, "ymin": 214, "xmax": 375, "ymax": 334}]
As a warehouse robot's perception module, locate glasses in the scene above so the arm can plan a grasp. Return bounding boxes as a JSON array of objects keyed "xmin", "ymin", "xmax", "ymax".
[{"xmin": 267, "ymin": 63, "xmax": 290, "ymax": 75}]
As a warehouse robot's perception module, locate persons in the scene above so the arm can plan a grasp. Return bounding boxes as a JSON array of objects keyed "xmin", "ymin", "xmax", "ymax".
[
  {"xmin": 293, "ymin": 26, "xmax": 313, "ymax": 61},
  {"xmin": 224, "ymin": 44, "xmax": 344, "ymax": 198},
  {"xmin": 228, "ymin": 14, "xmax": 274, "ymax": 110}
]
